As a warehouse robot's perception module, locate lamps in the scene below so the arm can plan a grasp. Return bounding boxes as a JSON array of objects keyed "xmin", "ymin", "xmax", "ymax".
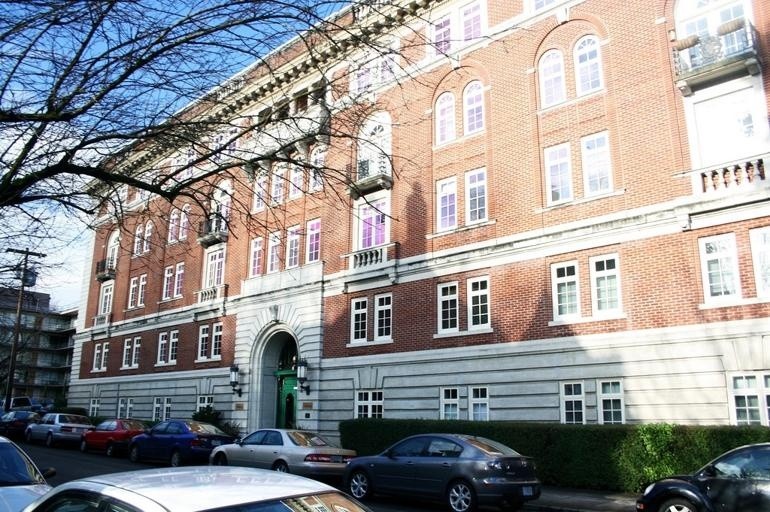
[
  {"xmin": 296, "ymin": 356, "xmax": 311, "ymax": 396},
  {"xmin": 229, "ymin": 362, "xmax": 242, "ymax": 397}
]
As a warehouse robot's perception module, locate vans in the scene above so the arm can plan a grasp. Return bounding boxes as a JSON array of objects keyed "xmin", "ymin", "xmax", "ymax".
[{"xmin": 2, "ymin": 396, "xmax": 34, "ymax": 412}]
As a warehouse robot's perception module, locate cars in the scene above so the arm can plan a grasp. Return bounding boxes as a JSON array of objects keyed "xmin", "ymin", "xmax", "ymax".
[
  {"xmin": 78, "ymin": 419, "xmax": 147, "ymax": 459},
  {"xmin": 208, "ymin": 428, "xmax": 358, "ymax": 485},
  {"xmin": 341, "ymin": 433, "xmax": 542, "ymax": 512},
  {"xmin": 127, "ymin": 418, "xmax": 236, "ymax": 467},
  {"xmin": 0, "ymin": 436, "xmax": 57, "ymax": 512},
  {"xmin": 635, "ymin": 442, "xmax": 770, "ymax": 512},
  {"xmin": 20, "ymin": 466, "xmax": 375, "ymax": 512},
  {"xmin": 23, "ymin": 412, "xmax": 96, "ymax": 447},
  {"xmin": 0, "ymin": 411, "xmax": 41, "ymax": 434}
]
